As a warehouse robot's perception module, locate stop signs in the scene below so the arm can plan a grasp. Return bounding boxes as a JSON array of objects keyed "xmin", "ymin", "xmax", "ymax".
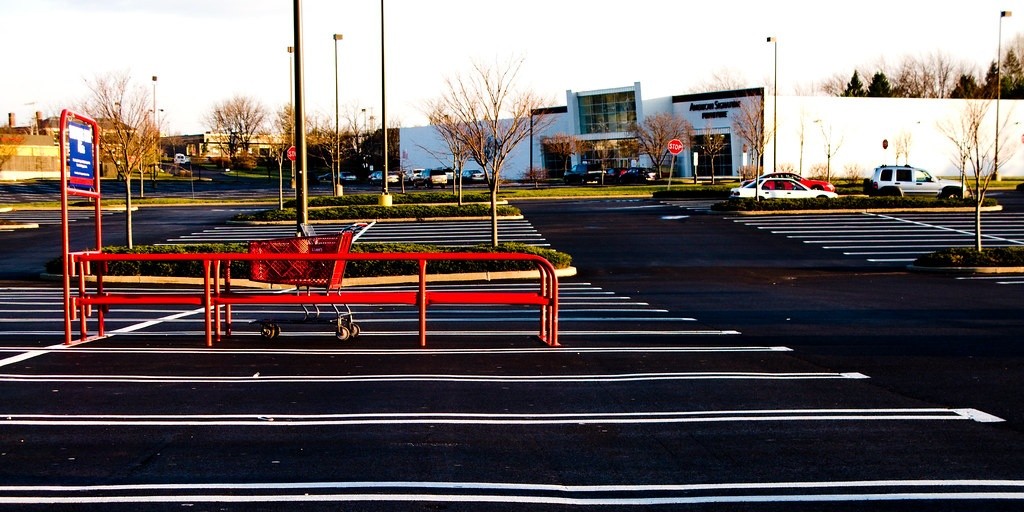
[
  {"xmin": 669, "ymin": 139, "xmax": 683, "ymax": 155},
  {"xmin": 286, "ymin": 146, "xmax": 296, "ymax": 162}
]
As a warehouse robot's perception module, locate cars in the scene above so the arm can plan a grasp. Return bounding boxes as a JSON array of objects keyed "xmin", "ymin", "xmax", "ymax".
[
  {"xmin": 318, "ymin": 171, "xmax": 357, "ymax": 185},
  {"xmin": 562, "ymin": 164, "xmax": 658, "ymax": 185},
  {"xmin": 367, "ymin": 169, "xmax": 487, "ymax": 189},
  {"xmin": 728, "ymin": 176, "xmax": 839, "ymax": 201},
  {"xmin": 739, "ymin": 172, "xmax": 836, "ymax": 193}
]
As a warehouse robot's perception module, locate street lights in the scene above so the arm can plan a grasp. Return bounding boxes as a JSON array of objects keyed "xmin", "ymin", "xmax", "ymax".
[
  {"xmin": 287, "ymin": 46, "xmax": 297, "ymax": 188},
  {"xmin": 333, "ymin": 33, "xmax": 343, "ymax": 198},
  {"xmin": 991, "ymin": 9, "xmax": 1013, "ymax": 180},
  {"xmin": 766, "ymin": 35, "xmax": 777, "ymax": 171},
  {"xmin": 152, "ymin": 75, "xmax": 157, "ymax": 142}
]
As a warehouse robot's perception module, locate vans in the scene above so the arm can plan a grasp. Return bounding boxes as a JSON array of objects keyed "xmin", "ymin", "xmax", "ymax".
[{"xmin": 173, "ymin": 153, "xmax": 186, "ymax": 165}]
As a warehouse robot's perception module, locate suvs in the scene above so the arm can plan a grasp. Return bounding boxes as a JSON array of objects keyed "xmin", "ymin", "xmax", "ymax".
[{"xmin": 862, "ymin": 165, "xmax": 968, "ymax": 200}]
